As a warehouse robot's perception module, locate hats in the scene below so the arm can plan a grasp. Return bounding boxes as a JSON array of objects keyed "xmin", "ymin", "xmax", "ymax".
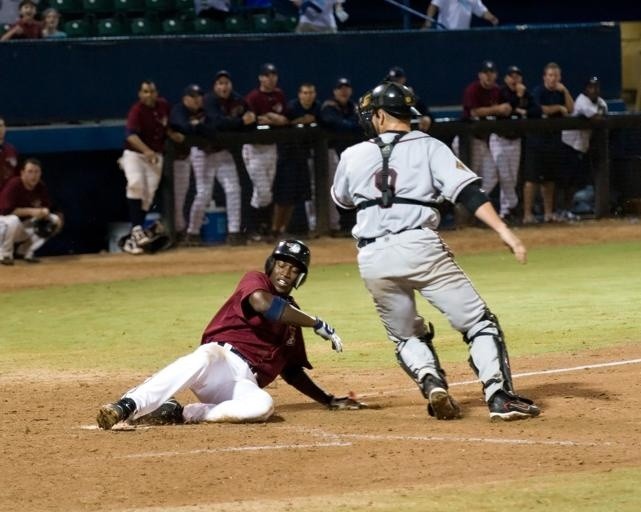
[
  {"xmin": 333, "ymin": 75, "xmax": 351, "ymax": 87},
  {"xmin": 384, "ymin": 66, "xmax": 407, "ymax": 80},
  {"xmin": 182, "ymin": 63, "xmax": 278, "ymax": 96},
  {"xmin": 478, "ymin": 60, "xmax": 522, "ymax": 75}
]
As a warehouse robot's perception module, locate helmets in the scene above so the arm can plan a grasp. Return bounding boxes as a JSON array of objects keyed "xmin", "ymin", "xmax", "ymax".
[
  {"xmin": 356, "ymin": 80, "xmax": 423, "ymax": 139},
  {"xmin": 30, "ymin": 213, "xmax": 62, "ymax": 238},
  {"xmin": 264, "ymin": 238, "xmax": 310, "ymax": 290}
]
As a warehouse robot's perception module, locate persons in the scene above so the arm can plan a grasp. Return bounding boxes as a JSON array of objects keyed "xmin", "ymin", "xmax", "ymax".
[
  {"xmin": 0, "ymin": 121, "xmax": 63, "ymax": 266},
  {"xmin": 332, "ymin": 80, "xmax": 544, "ymax": 419},
  {"xmin": 464, "ymin": 58, "xmax": 610, "ymax": 228},
  {"xmin": 422, "ymin": 0, "xmax": 499, "ymax": 30},
  {"xmin": 96, "ymin": 239, "xmax": 369, "ymax": 429},
  {"xmin": 270, "ymin": 0, "xmax": 350, "ymax": 35},
  {"xmin": 118, "ymin": 63, "xmax": 434, "ymax": 255},
  {"xmin": 1, "ymin": 2, "xmax": 66, "ymax": 40}
]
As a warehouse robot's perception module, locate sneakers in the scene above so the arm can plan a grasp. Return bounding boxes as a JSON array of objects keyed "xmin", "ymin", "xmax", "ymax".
[
  {"xmin": 487, "ymin": 389, "xmax": 541, "ymax": 423},
  {"xmin": 97, "ymin": 397, "xmax": 136, "ymax": 430},
  {"xmin": 422, "ymin": 374, "xmax": 460, "ymax": 419},
  {"xmin": 128, "ymin": 396, "xmax": 183, "ymax": 425}
]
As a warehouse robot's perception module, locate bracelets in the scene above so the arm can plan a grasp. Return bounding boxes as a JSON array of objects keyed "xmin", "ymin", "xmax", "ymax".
[{"xmin": 262, "ymin": 296, "xmax": 289, "ymax": 325}]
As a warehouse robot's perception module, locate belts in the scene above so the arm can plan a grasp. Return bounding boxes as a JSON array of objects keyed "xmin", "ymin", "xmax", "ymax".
[{"xmin": 218, "ymin": 342, "xmax": 259, "ymax": 380}]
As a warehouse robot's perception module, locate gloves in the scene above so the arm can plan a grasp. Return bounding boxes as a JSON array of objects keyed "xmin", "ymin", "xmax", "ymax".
[
  {"xmin": 312, "ymin": 316, "xmax": 344, "ymax": 353},
  {"xmin": 329, "ymin": 397, "xmax": 368, "ymax": 410}
]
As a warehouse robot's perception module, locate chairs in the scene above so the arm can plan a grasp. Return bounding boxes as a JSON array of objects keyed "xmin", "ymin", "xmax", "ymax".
[{"xmin": 42, "ymin": 1, "xmax": 299, "ymax": 35}]
